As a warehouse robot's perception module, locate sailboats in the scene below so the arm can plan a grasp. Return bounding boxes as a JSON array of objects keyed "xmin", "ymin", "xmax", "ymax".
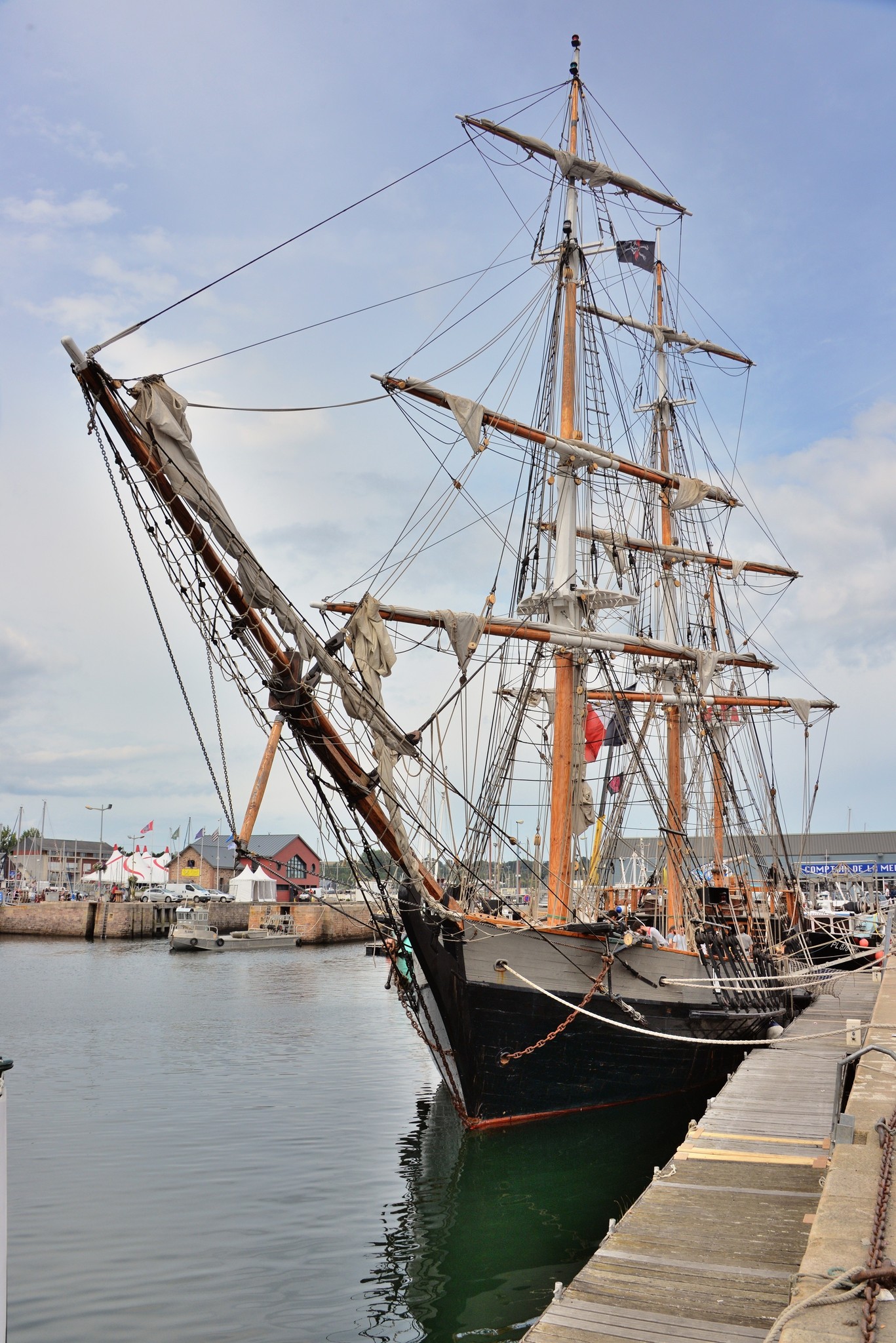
[
  {"xmin": 317, "ymin": 766, "xmax": 896, "ymax": 974},
  {"xmin": 0, "ymin": 799, "xmax": 77, "ymax": 907},
  {"xmin": 59, "ymin": 30, "xmax": 892, "ymax": 1130}
]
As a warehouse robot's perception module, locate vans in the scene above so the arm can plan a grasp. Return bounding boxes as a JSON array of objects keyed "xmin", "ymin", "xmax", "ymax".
[
  {"xmin": 299, "ymin": 887, "xmax": 322, "ymax": 902},
  {"xmin": 165, "ymin": 883, "xmax": 211, "ymax": 903}
]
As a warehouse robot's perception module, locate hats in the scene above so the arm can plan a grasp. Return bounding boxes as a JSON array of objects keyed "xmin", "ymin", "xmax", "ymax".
[
  {"xmin": 671, "ymin": 926, "xmax": 675, "ymax": 929},
  {"xmin": 715, "ymin": 931, "xmax": 719, "ymax": 935}
]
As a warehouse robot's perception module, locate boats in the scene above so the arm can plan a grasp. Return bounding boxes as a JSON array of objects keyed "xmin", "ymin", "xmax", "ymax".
[{"xmin": 167, "ymin": 893, "xmax": 308, "ymax": 951}]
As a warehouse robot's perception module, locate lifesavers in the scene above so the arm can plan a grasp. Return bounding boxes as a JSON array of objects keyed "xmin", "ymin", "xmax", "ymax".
[
  {"xmin": 296, "ymin": 939, "xmax": 302, "ymax": 945},
  {"xmin": 381, "ymin": 918, "xmax": 391, "ymax": 928},
  {"xmin": 190, "ymin": 938, "xmax": 198, "ymax": 945},
  {"xmin": 217, "ymin": 938, "xmax": 224, "ymax": 946}
]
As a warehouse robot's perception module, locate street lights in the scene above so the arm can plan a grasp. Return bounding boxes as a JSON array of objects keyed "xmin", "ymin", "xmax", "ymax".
[
  {"xmin": 127, "ymin": 835, "xmax": 145, "ymax": 876},
  {"xmin": 516, "ymin": 820, "xmax": 524, "ymax": 903},
  {"xmin": 506, "ymin": 866, "xmax": 511, "ymax": 888},
  {"xmin": 493, "ymin": 843, "xmax": 498, "ymax": 883},
  {"xmin": 85, "ymin": 803, "xmax": 112, "ymax": 902},
  {"xmin": 579, "ymin": 837, "xmax": 588, "ymax": 872}
]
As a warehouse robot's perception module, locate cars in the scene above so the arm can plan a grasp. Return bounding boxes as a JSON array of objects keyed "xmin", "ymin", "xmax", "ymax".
[
  {"xmin": 68, "ymin": 890, "xmax": 88, "ymax": 898},
  {"xmin": 204, "ymin": 889, "xmax": 235, "ymax": 903},
  {"xmin": 140, "ymin": 888, "xmax": 183, "ymax": 903}
]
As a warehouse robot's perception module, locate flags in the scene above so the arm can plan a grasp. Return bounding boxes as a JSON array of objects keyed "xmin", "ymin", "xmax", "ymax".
[
  {"xmin": 616, "ymin": 239, "xmax": 656, "ymax": 273},
  {"xmin": 603, "ymin": 682, "xmax": 739, "ymax": 796},
  {"xmin": 141, "ymin": 820, "xmax": 234, "ymax": 843}
]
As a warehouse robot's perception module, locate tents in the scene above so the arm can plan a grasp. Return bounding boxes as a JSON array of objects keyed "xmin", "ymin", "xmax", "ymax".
[{"xmin": 229, "ymin": 864, "xmax": 278, "ymax": 902}]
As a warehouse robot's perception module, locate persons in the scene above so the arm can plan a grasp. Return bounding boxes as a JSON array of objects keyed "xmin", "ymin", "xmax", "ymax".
[
  {"xmin": 5, "ymin": 885, "xmax": 126, "ymax": 903},
  {"xmin": 603, "ymin": 910, "xmax": 753, "ymax": 959},
  {"xmin": 293, "ymin": 888, "xmax": 313, "ymax": 902},
  {"xmin": 769, "ymin": 864, "xmax": 896, "ymax": 912}
]
